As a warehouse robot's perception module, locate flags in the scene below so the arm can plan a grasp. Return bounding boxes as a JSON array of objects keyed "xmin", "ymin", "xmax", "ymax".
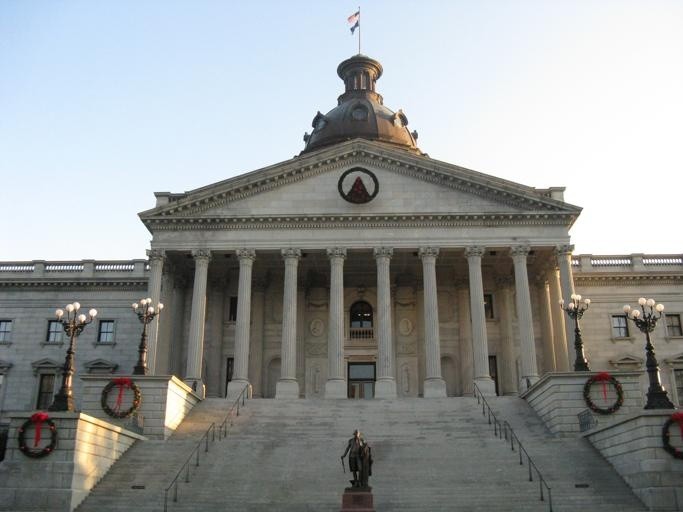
[{"xmin": 347, "ymin": 10, "xmax": 358, "ymax": 34}]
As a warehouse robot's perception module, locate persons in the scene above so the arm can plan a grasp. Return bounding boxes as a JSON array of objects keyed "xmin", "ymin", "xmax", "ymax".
[
  {"xmin": 338, "ymin": 428, "xmax": 363, "ymax": 482},
  {"xmin": 358, "ymin": 439, "xmax": 373, "ymax": 486}
]
